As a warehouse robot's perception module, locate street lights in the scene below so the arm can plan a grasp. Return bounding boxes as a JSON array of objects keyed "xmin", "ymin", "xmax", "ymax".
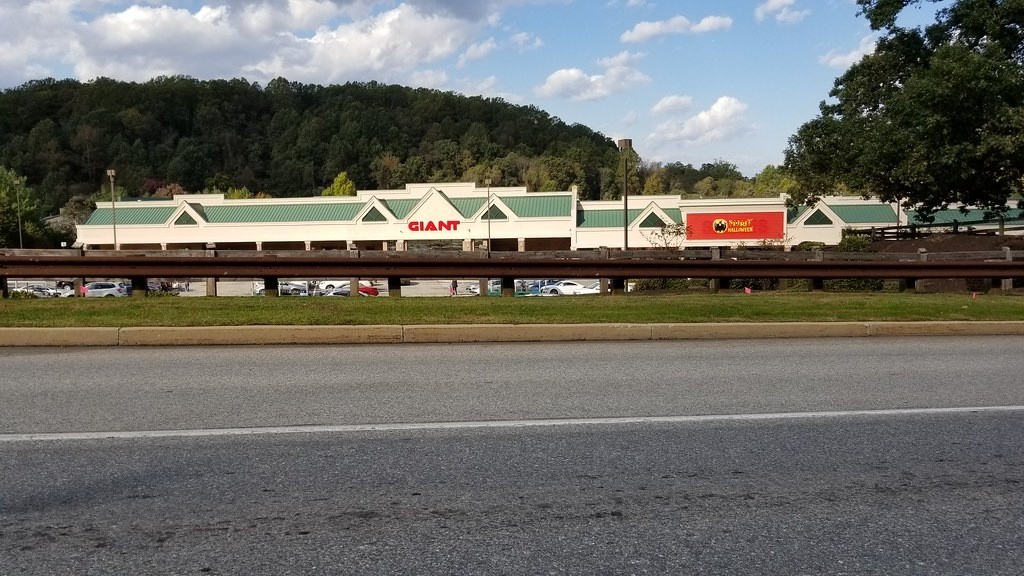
[
  {"xmin": 618, "ymin": 138, "xmax": 632, "ymax": 251},
  {"xmin": 13, "ymin": 179, "xmax": 23, "ymax": 250},
  {"xmin": 107, "ymin": 169, "xmax": 119, "ymax": 251},
  {"xmin": 484, "ymin": 178, "xmax": 492, "ymax": 253}
]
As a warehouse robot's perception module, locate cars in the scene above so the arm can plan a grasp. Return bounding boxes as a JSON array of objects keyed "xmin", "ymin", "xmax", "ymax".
[
  {"xmin": 250, "ymin": 279, "xmax": 386, "ymax": 298},
  {"xmin": 13, "ymin": 278, "xmax": 182, "ymax": 297},
  {"xmin": 466, "ymin": 279, "xmax": 636, "ymax": 298}
]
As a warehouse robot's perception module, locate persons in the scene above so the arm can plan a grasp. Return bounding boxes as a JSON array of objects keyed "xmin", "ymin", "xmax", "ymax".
[
  {"xmin": 451, "ymin": 280, "xmax": 458, "ymax": 295},
  {"xmin": 184, "ymin": 278, "xmax": 191, "ymax": 292}
]
